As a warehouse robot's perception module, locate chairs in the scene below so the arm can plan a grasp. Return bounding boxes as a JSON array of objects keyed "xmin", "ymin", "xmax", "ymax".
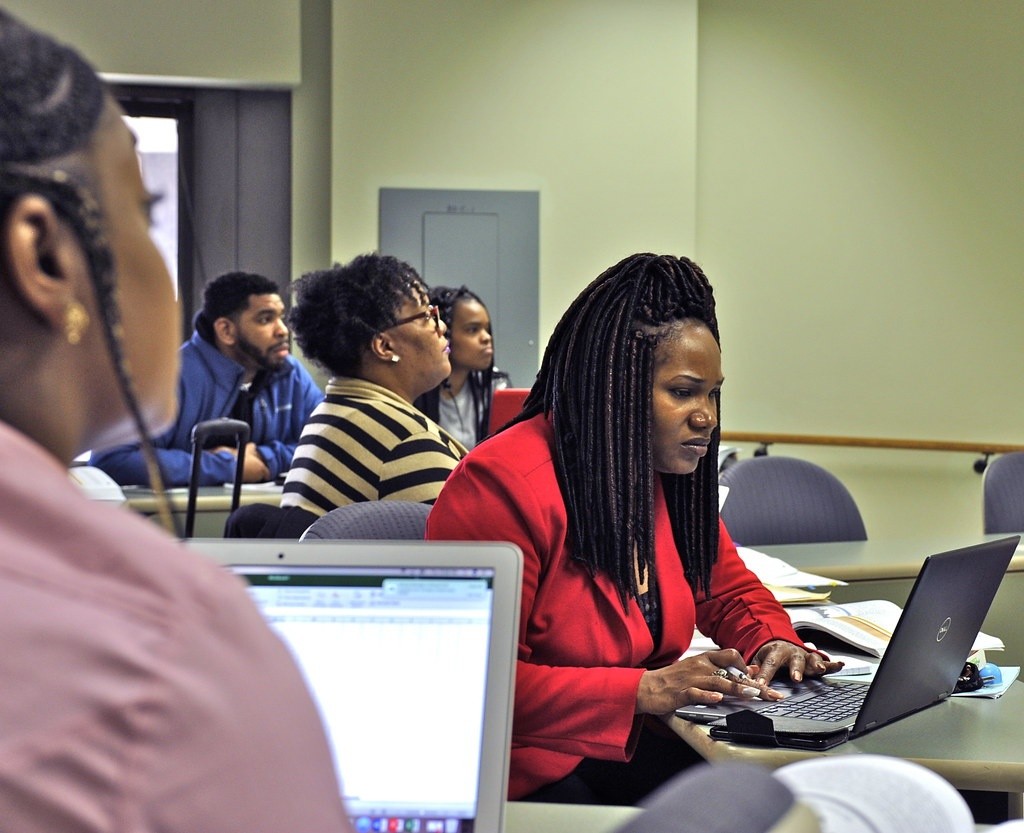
[
  {"xmin": 298, "ymin": 500, "xmax": 433, "ymax": 544},
  {"xmin": 981, "ymin": 453, "xmax": 1024, "ymax": 534},
  {"xmin": 717, "ymin": 455, "xmax": 871, "ymax": 547}
]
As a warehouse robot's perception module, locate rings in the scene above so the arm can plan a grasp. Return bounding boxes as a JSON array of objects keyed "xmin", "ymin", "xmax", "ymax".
[{"xmin": 711, "ymin": 668, "xmax": 728, "ymax": 679}]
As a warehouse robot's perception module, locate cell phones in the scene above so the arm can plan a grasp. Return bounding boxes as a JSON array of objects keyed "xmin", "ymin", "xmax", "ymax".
[{"xmin": 708, "ymin": 708, "xmax": 849, "ymax": 752}]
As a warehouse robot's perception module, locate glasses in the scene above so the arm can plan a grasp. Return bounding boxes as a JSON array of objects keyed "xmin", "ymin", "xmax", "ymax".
[{"xmin": 363, "ymin": 305, "xmax": 440, "ymax": 351}]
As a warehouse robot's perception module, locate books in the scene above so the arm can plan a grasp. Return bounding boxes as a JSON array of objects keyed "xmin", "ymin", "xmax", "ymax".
[{"xmin": 721, "ymin": 540, "xmax": 1004, "ymax": 659}]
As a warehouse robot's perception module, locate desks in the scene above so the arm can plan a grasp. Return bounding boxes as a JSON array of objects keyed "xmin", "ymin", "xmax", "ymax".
[
  {"xmin": 656, "ymin": 647, "xmax": 1024, "ymax": 821},
  {"xmin": 121, "ymin": 479, "xmax": 284, "ymax": 538},
  {"xmin": 735, "ymin": 531, "xmax": 1024, "ymax": 686}
]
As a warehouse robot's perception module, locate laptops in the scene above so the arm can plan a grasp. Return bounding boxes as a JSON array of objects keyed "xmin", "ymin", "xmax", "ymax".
[
  {"xmin": 176, "ymin": 539, "xmax": 524, "ymax": 833},
  {"xmin": 678, "ymin": 534, "xmax": 1022, "ymax": 738}
]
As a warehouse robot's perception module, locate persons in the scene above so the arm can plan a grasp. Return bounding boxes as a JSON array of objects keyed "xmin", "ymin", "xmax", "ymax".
[
  {"xmin": 89, "ymin": 273, "xmax": 327, "ymax": 489},
  {"xmin": 428, "ymin": 251, "xmax": 847, "ymax": 800},
  {"xmin": 412, "ymin": 286, "xmax": 512, "ymax": 450},
  {"xmin": 0, "ymin": 10, "xmax": 349, "ymax": 833},
  {"xmin": 278, "ymin": 252, "xmax": 472, "ymax": 508}
]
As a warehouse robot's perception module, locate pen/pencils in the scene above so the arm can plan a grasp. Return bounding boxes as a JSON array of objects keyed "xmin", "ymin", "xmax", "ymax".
[{"xmin": 725, "ymin": 665, "xmax": 746, "ymax": 680}]
